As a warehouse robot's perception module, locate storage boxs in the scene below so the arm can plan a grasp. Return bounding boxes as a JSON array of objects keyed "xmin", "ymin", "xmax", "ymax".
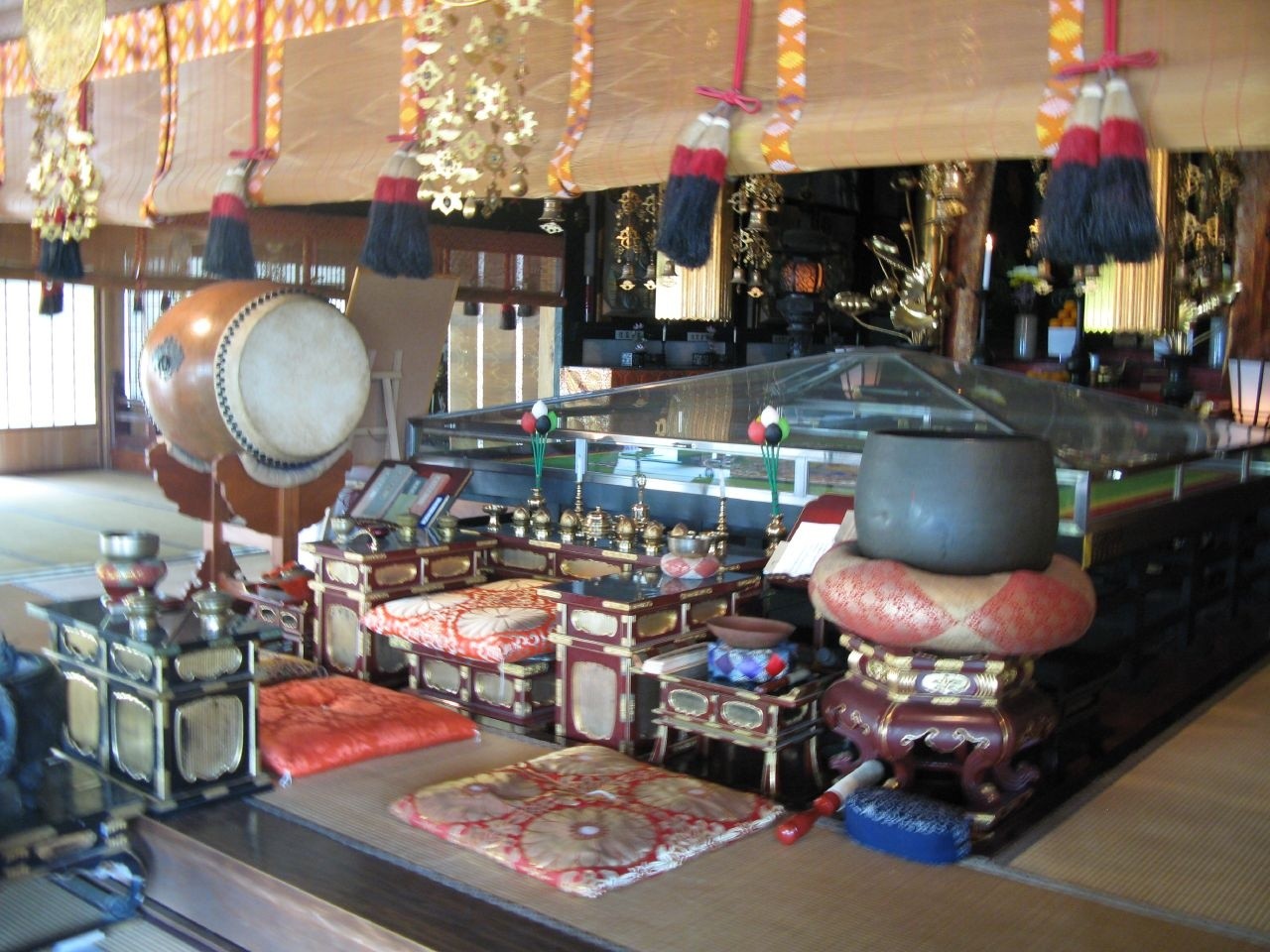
[{"xmin": 24, "ymin": 590, "xmax": 276, "ymax": 815}]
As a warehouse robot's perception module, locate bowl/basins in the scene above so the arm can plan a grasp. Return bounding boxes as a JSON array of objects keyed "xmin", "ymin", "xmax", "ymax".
[
  {"xmin": 854, "ymin": 428, "xmax": 1059, "ymax": 575},
  {"xmin": 667, "ymin": 535, "xmax": 714, "ymax": 558}
]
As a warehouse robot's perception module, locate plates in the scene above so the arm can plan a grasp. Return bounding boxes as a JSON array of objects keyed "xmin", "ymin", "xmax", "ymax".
[{"xmin": 708, "ymin": 617, "xmax": 796, "ymax": 649}]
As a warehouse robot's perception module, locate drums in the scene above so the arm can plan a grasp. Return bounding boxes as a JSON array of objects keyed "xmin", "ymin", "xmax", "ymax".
[{"xmin": 135, "ymin": 278, "xmax": 374, "ymax": 474}]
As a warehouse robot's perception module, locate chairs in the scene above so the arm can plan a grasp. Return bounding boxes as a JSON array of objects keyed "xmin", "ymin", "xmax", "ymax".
[{"xmin": 309, "ymin": 518, "xmax": 762, "ymax": 758}]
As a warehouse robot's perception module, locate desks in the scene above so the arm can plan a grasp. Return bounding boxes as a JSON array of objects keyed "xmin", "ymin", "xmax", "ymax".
[{"xmin": 629, "ymin": 659, "xmax": 848, "ymax": 804}]
